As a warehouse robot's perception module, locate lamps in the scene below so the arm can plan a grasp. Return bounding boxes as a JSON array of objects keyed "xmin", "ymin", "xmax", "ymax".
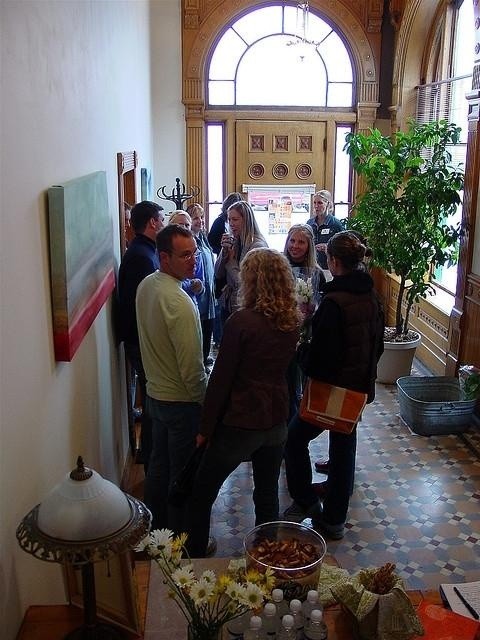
[
  {"xmin": 285, "ymin": 0, "xmax": 320, "ymax": 62},
  {"xmin": 16, "ymin": 454, "xmax": 153, "ymax": 639}
]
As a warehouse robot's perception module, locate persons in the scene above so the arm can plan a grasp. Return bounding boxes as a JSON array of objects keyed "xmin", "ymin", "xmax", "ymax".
[{"xmin": 112, "ymin": 187, "xmax": 386, "ymax": 559}]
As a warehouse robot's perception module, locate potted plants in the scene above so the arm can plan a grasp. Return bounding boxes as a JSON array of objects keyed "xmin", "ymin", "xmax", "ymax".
[{"xmin": 340, "ymin": 114, "xmax": 469, "ymax": 384}]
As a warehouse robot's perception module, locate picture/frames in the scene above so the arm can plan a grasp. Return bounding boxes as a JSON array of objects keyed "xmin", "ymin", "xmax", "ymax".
[{"xmin": 54, "ymin": 525, "xmax": 146, "ymax": 640}]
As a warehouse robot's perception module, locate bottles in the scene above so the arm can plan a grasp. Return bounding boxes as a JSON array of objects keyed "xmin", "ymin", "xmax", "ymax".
[{"xmin": 226, "ymin": 590, "xmax": 328, "ymax": 639}]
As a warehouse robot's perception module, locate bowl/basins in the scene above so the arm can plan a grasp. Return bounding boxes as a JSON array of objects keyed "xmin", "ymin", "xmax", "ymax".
[{"xmin": 242, "ymin": 521, "xmax": 328, "ymax": 603}]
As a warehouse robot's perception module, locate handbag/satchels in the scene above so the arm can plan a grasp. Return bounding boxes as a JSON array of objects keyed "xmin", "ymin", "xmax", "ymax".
[{"xmin": 299, "ymin": 377, "xmax": 368, "ymax": 435}]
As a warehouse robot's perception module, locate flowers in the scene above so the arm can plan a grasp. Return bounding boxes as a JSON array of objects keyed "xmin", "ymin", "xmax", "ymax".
[
  {"xmin": 135, "ymin": 526, "xmax": 276, "ymax": 640},
  {"xmin": 295, "ymin": 277, "xmax": 317, "ymax": 344}
]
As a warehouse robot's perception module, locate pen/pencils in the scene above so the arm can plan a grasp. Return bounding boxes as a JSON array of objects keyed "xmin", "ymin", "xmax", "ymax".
[{"xmin": 454, "ymin": 587, "xmax": 479, "ymax": 619}]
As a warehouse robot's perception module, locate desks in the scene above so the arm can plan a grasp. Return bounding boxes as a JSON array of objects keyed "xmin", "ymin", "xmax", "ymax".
[{"xmin": 116, "ymin": 555, "xmax": 480, "ymax": 639}]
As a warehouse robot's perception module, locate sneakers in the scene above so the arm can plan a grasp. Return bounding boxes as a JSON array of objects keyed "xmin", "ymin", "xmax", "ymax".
[
  {"xmin": 207, "ymin": 537, "xmax": 216, "ymax": 557},
  {"xmin": 282, "ymin": 459, "xmax": 347, "ymax": 539}
]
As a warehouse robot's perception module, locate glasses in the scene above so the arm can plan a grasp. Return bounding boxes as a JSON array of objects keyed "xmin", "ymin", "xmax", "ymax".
[{"xmin": 171, "ymin": 250, "xmax": 202, "ymax": 261}]
